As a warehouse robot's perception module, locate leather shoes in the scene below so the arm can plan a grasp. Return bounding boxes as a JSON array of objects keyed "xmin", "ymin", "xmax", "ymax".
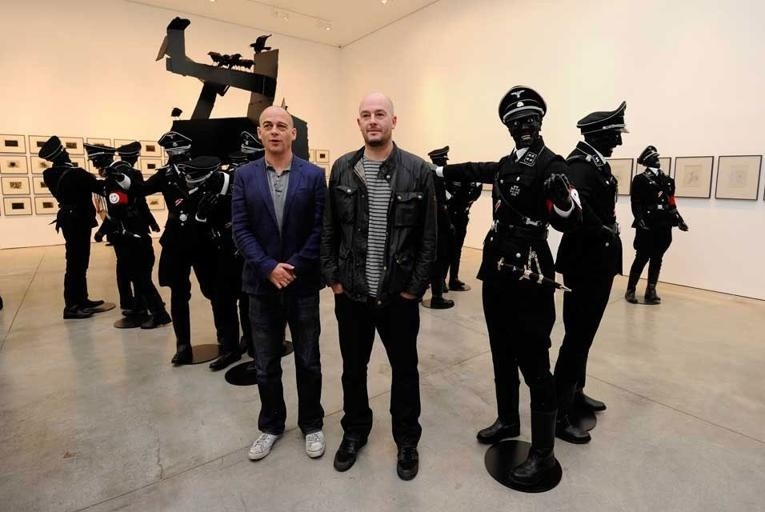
[
  {"xmin": 333, "ymin": 432, "xmax": 367, "ymax": 471},
  {"xmin": 396, "ymin": 441, "xmax": 420, "ymax": 481}
]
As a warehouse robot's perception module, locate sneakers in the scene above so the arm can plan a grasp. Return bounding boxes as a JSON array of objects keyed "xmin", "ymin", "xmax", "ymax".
[
  {"xmin": 247, "ymin": 431, "xmax": 284, "ymax": 461},
  {"xmin": 305, "ymin": 432, "xmax": 326, "ymax": 459}
]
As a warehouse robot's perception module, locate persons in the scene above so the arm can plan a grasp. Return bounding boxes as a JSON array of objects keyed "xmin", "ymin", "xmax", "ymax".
[
  {"xmin": 38, "ymin": 136, "xmax": 107, "ymax": 319},
  {"xmin": 326, "ymin": 92, "xmax": 439, "ymax": 480},
  {"xmin": 230, "ymin": 105, "xmax": 328, "ymax": 462},
  {"xmin": 82, "ymin": 140, "xmax": 170, "ymax": 330},
  {"xmin": 625, "ymin": 144, "xmax": 688, "ymax": 304},
  {"xmin": 553, "ymin": 97, "xmax": 631, "ymax": 444},
  {"xmin": 143, "ymin": 132, "xmax": 265, "ymax": 371},
  {"xmin": 430, "ymin": 84, "xmax": 582, "ymax": 478},
  {"xmin": 427, "ymin": 146, "xmax": 484, "ymax": 310}
]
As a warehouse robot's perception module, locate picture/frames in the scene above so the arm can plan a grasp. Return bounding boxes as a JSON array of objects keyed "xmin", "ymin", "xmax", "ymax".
[
  {"xmin": 3, "ymin": 197, "xmax": 33, "ymax": 215},
  {"xmin": 1, "ymin": 156, "xmax": 28, "ymax": 174},
  {"xmin": 635, "ymin": 156, "xmax": 672, "ymax": 196},
  {"xmin": 34, "ymin": 196, "xmax": 60, "ymax": 217},
  {"xmin": 1, "ymin": 134, "xmax": 27, "ymax": 154},
  {"xmin": 314, "ymin": 149, "xmax": 330, "ymax": 164},
  {"xmin": 606, "ymin": 157, "xmax": 634, "ymax": 196},
  {"xmin": 29, "ymin": 135, "xmax": 51, "ymax": 154},
  {"xmin": 32, "ymin": 176, "xmax": 54, "ymax": 195},
  {"xmin": 69, "ymin": 157, "xmax": 86, "ymax": 171},
  {"xmin": 58, "ymin": 137, "xmax": 84, "ymax": 155},
  {"xmin": 674, "ymin": 156, "xmax": 715, "ymax": 199},
  {"xmin": 139, "ymin": 158, "xmax": 162, "ymax": 176},
  {"xmin": 30, "ymin": 156, "xmax": 53, "ymax": 175},
  {"xmin": 2, "ymin": 177, "xmax": 31, "ymax": 196},
  {"xmin": 87, "ymin": 137, "xmax": 112, "ymax": 147},
  {"xmin": 145, "ymin": 193, "xmax": 166, "ymax": 210},
  {"xmin": 114, "ymin": 139, "xmax": 137, "ymax": 154},
  {"xmin": 114, "ymin": 156, "xmax": 139, "ymax": 170},
  {"xmin": 714, "ymin": 155, "xmax": 763, "ymax": 200},
  {"xmin": 139, "ymin": 140, "xmax": 162, "ymax": 157},
  {"xmin": 89, "ymin": 159, "xmax": 100, "ymax": 175}
]
